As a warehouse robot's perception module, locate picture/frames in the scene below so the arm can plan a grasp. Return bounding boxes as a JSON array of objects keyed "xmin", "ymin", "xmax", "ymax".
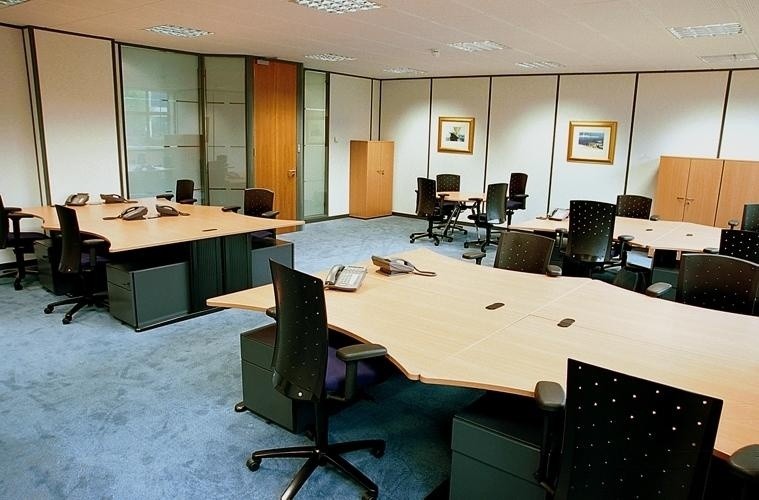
[
  {"xmin": 567, "ymin": 120, "xmax": 618, "ymax": 165},
  {"xmin": 437, "ymin": 116, "xmax": 475, "ymax": 155}
]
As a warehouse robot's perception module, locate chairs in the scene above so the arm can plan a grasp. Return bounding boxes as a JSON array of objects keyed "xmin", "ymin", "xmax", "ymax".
[
  {"xmin": 44, "ymin": 204, "xmax": 108, "ymax": 325},
  {"xmin": 156, "ymin": 179, "xmax": 197, "ymax": 205},
  {"xmin": 535, "ymin": 358, "xmax": 758, "ymax": 498},
  {"xmin": 221, "ymin": 187, "xmax": 280, "ymax": 239},
  {"xmin": 247, "ymin": 258, "xmax": 387, "ymax": 500},
  {"xmin": 0, "ymin": 195, "xmax": 49, "ymax": 289}
]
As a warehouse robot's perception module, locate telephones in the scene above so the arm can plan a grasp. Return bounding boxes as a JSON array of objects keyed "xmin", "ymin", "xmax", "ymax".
[
  {"xmin": 100, "ymin": 193, "xmax": 125, "ymax": 204},
  {"xmin": 156, "ymin": 203, "xmax": 179, "ymax": 216},
  {"xmin": 546, "ymin": 207, "xmax": 570, "ymax": 221},
  {"xmin": 323, "ymin": 263, "xmax": 367, "ymax": 292},
  {"xmin": 117, "ymin": 205, "xmax": 148, "ymax": 220},
  {"xmin": 371, "ymin": 255, "xmax": 415, "ymax": 275},
  {"xmin": 65, "ymin": 192, "xmax": 89, "ymax": 206}
]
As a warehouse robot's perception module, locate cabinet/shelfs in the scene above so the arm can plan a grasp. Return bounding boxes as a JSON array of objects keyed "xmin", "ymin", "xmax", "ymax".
[
  {"xmin": 349, "ymin": 140, "xmax": 394, "ymax": 219},
  {"xmin": 654, "ymin": 156, "xmax": 759, "ymax": 230}
]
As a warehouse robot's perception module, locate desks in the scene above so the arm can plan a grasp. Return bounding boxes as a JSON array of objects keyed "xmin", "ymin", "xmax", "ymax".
[
  {"xmin": 16, "ymin": 197, "xmax": 305, "ymax": 332},
  {"xmin": 205, "ymin": 246, "xmax": 758, "ymax": 460}
]
